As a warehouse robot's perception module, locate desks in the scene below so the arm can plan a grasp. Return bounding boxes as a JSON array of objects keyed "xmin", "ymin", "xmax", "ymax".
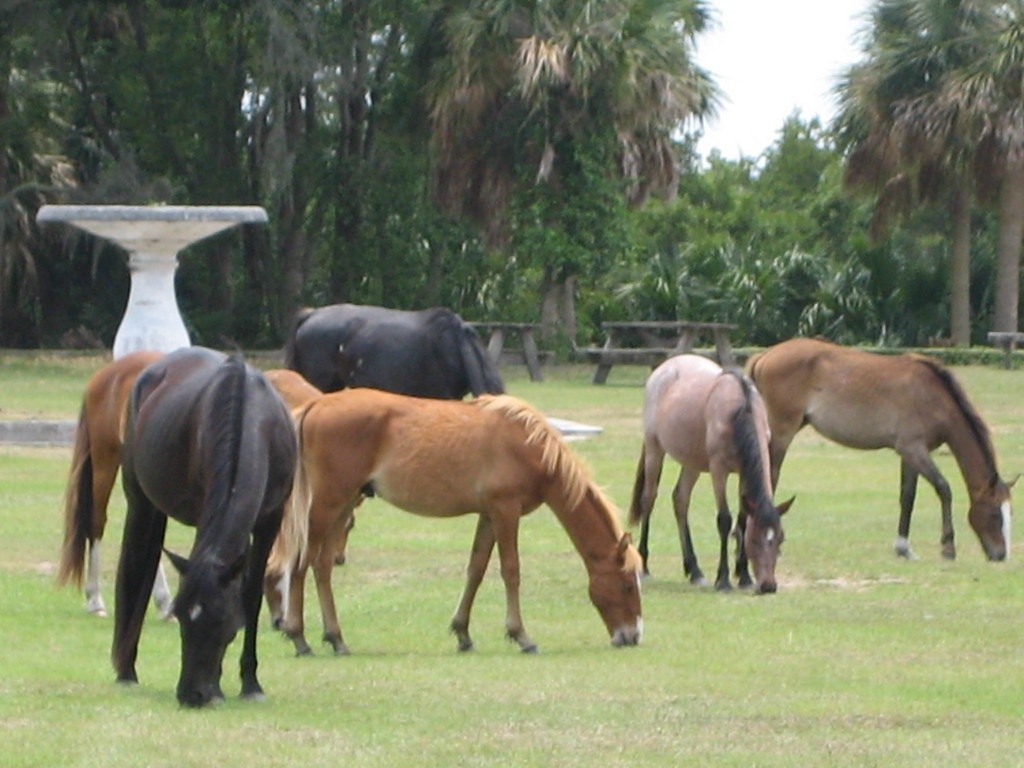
[
  {"xmin": 987, "ymin": 332, "xmax": 1024, "ymax": 370},
  {"xmin": 470, "ymin": 323, "xmax": 545, "ymax": 382},
  {"xmin": 594, "ymin": 323, "xmax": 740, "ymax": 385}
]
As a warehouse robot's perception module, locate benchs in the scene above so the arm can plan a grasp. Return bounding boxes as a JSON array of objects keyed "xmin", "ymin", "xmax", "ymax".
[
  {"xmin": 502, "ymin": 349, "xmax": 555, "ymax": 358},
  {"xmin": 585, "ymin": 348, "xmax": 757, "ymax": 362}
]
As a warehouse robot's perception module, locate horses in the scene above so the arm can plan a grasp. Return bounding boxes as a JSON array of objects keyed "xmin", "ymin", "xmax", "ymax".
[
  {"xmin": 745, "ymin": 337, "xmax": 1023, "ymax": 563},
  {"xmin": 56, "ymin": 302, "xmax": 645, "ymax": 711},
  {"xmin": 627, "ymin": 351, "xmax": 798, "ymax": 595}
]
